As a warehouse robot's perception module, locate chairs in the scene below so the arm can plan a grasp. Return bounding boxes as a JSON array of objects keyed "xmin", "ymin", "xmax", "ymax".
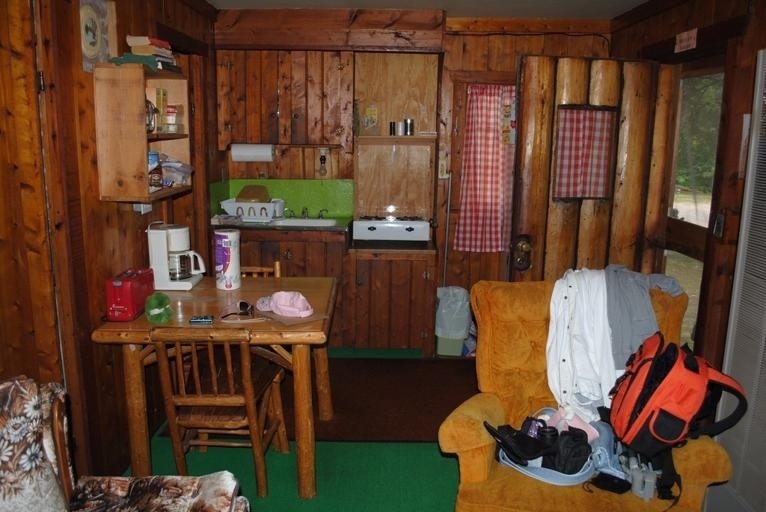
[
  {"xmin": 0, "ymin": 374, "xmax": 251, "ymax": 512},
  {"xmin": 151, "ymin": 327, "xmax": 291, "ymax": 498},
  {"xmin": 241, "ymin": 261, "xmax": 282, "ymax": 277},
  {"xmin": 437, "ymin": 280, "xmax": 734, "ymax": 512}
]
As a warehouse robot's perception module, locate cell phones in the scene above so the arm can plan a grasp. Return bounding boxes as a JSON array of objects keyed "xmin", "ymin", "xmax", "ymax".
[{"xmin": 190, "ymin": 316, "xmax": 212, "ymax": 322}]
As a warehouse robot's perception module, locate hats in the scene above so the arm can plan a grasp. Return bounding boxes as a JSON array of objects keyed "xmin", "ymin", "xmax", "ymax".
[{"xmin": 256, "ymin": 291, "xmax": 315, "ymax": 318}]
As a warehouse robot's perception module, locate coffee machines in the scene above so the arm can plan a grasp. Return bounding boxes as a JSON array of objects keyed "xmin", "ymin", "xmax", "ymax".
[{"xmin": 147, "ymin": 221, "xmax": 206, "ymax": 292}]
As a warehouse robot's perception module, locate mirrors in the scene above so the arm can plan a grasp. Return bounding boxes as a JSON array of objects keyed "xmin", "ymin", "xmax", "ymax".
[{"xmin": 551, "ymin": 104, "xmax": 621, "ymax": 201}]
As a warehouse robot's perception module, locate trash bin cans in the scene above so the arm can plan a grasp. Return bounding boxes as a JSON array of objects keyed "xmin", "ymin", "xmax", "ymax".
[{"xmin": 435, "ymin": 286, "xmax": 471, "ymax": 356}]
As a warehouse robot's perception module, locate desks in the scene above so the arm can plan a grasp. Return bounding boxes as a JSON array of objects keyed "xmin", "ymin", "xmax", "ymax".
[{"xmin": 91, "ymin": 277, "xmax": 339, "ymax": 499}]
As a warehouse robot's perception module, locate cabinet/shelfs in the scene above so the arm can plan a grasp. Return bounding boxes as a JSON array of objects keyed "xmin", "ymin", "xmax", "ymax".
[
  {"xmin": 91, "ymin": 62, "xmax": 194, "ymax": 205},
  {"xmin": 348, "ymin": 250, "xmax": 438, "ymax": 358},
  {"xmin": 209, "ymin": 229, "xmax": 348, "ymax": 358},
  {"xmin": 215, "ymin": 50, "xmax": 356, "ymax": 153}
]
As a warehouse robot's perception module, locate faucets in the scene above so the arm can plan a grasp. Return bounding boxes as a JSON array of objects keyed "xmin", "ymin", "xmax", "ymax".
[{"xmin": 301, "ymin": 207, "xmax": 310, "ymax": 220}]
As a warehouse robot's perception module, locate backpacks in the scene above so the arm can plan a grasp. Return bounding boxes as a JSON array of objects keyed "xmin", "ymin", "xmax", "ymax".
[{"xmin": 610, "ymin": 333, "xmax": 748, "ymax": 458}]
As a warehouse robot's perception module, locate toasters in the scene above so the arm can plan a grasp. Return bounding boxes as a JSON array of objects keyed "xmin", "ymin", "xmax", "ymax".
[{"xmin": 105, "ymin": 267, "xmax": 154, "ymax": 321}]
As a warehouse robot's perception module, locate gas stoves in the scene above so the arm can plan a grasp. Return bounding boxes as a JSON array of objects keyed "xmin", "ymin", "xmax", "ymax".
[{"xmin": 352, "ymin": 216, "xmax": 431, "ymax": 248}]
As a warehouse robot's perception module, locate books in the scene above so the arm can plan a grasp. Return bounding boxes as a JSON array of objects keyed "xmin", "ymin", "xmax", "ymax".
[{"xmin": 124, "ymin": 35, "xmax": 184, "ymax": 134}]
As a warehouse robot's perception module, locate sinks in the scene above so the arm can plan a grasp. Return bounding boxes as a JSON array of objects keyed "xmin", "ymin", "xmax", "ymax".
[{"xmin": 272, "ymin": 218, "xmax": 337, "ymax": 227}]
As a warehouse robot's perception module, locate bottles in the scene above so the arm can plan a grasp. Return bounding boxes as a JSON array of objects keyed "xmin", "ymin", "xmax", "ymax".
[{"xmin": 390, "ymin": 119, "xmax": 414, "ymax": 136}]
